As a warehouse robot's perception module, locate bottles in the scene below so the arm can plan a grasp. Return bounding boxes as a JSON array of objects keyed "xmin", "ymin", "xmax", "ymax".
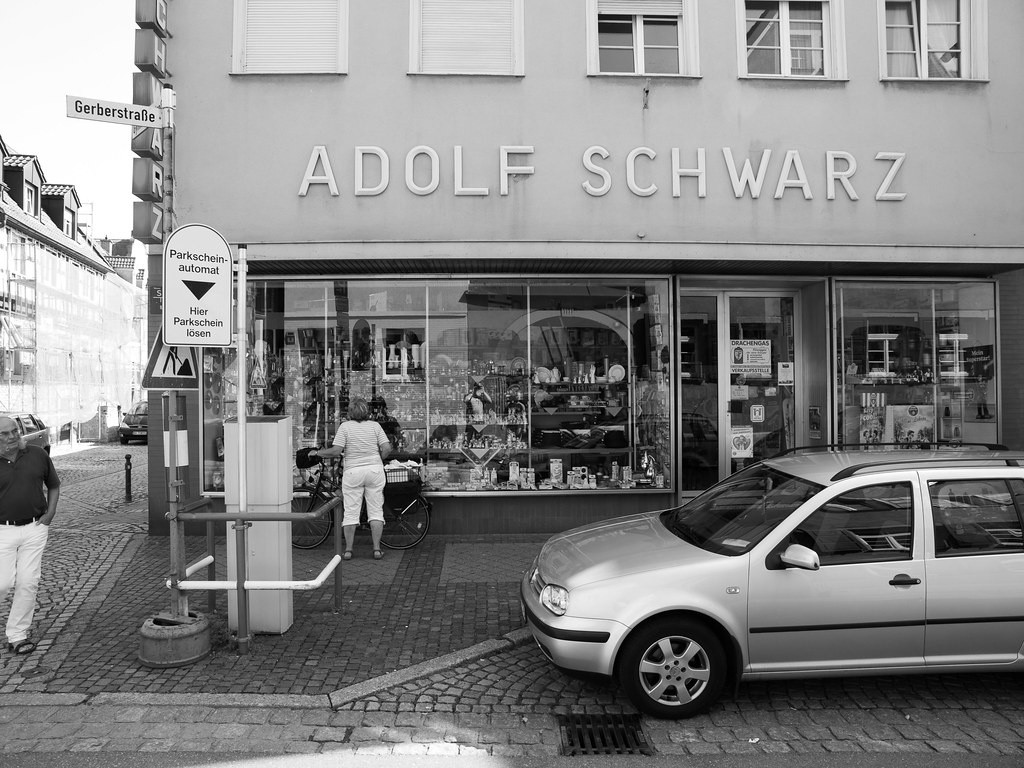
[
  {"xmin": 597, "ymin": 462, "xmax": 631, "ymax": 488},
  {"xmin": 491, "ymin": 468, "xmax": 497, "ymax": 484},
  {"xmin": 430, "ymin": 359, "xmax": 508, "ymax": 376},
  {"xmin": 430, "ymin": 433, "xmax": 528, "ymax": 449},
  {"xmin": 655, "ymin": 474, "xmax": 664, "ymax": 488},
  {"xmin": 484, "ymin": 467, "xmax": 490, "ymax": 484},
  {"xmin": 510, "ymin": 366, "xmax": 525, "ymax": 376}
]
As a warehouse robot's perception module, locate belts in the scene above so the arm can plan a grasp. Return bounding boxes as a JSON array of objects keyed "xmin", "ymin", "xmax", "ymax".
[{"xmin": 0, "ymin": 512, "xmax": 45, "ymax": 527}]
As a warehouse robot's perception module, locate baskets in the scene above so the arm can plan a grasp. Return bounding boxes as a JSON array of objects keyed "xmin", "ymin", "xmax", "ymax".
[{"xmin": 384, "ymin": 466, "xmax": 420, "ymax": 483}]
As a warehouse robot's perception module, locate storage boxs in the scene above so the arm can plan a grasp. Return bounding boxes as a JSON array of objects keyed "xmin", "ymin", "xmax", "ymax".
[{"xmin": 445, "ymin": 294, "xmax": 665, "ymax": 489}]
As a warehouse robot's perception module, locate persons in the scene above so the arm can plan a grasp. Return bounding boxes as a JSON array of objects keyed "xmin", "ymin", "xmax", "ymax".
[
  {"xmin": 308, "ymin": 396, "xmax": 392, "ymax": 560},
  {"xmin": 367, "ymin": 396, "xmax": 408, "ymax": 451},
  {"xmin": 0, "ymin": 416, "xmax": 61, "ymax": 654}
]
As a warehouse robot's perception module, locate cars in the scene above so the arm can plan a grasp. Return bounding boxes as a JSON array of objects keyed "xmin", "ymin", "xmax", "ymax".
[
  {"xmin": 0, "ymin": 412, "xmax": 50, "ymax": 458},
  {"xmin": 517, "ymin": 412, "xmax": 737, "ymax": 490},
  {"xmin": 116, "ymin": 401, "xmax": 148, "ymax": 445},
  {"xmin": 520, "ymin": 442, "xmax": 1024, "ymax": 720}
]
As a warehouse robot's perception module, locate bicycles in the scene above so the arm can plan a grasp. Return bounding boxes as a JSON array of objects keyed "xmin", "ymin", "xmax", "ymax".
[{"xmin": 292, "ymin": 445, "xmax": 434, "ymax": 550}]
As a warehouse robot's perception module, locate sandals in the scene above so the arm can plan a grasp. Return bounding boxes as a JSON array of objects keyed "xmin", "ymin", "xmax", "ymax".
[
  {"xmin": 344, "ymin": 551, "xmax": 352, "ymax": 560},
  {"xmin": 9, "ymin": 639, "xmax": 35, "ymax": 654},
  {"xmin": 373, "ymin": 549, "xmax": 385, "ymax": 559}
]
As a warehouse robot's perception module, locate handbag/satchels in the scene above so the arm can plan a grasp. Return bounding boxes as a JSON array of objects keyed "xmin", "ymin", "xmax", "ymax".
[
  {"xmin": 385, "ymin": 480, "xmax": 421, "ymax": 514},
  {"xmin": 296, "ymin": 447, "xmax": 322, "ymax": 469}
]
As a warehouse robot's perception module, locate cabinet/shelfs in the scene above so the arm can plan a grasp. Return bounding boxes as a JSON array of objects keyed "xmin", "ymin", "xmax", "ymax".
[
  {"xmin": 867, "ymin": 334, "xmax": 899, "ymax": 378},
  {"xmin": 325, "ymin": 368, "xmax": 656, "ymax": 474},
  {"xmin": 918, "ymin": 333, "xmax": 969, "ymax": 377}
]
